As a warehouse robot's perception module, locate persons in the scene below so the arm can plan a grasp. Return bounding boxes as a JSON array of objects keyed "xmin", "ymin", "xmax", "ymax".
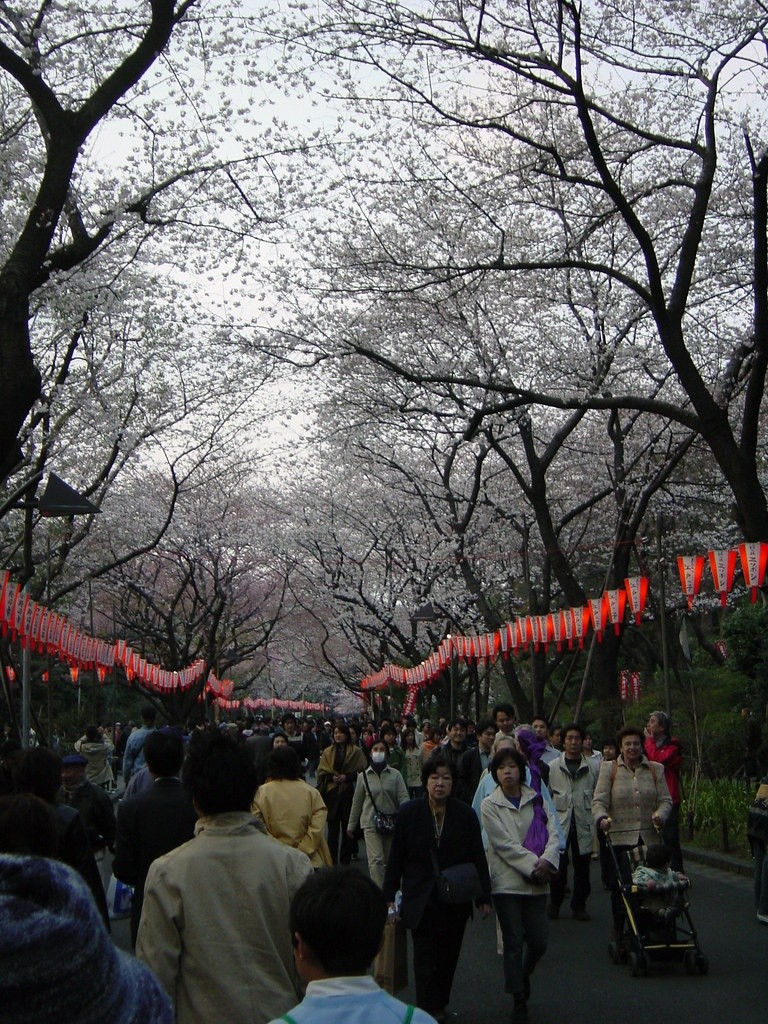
[
  {"xmin": 745, "ymin": 773, "xmax": 768, "ymax": 922},
  {"xmin": 641, "ymin": 712, "xmax": 686, "ymax": 874},
  {"xmin": 382, "ymin": 753, "xmax": 492, "ymax": 1024},
  {"xmin": 479, "ymin": 747, "xmax": 559, "ymax": 1022},
  {"xmin": 204, "ymin": 711, "xmax": 333, "ymax": 872},
  {"xmin": 304, "ymin": 714, "xmax": 425, "ymax": 892},
  {"xmin": 74, "ymin": 706, "xmax": 205, "ymax": 791},
  {"xmin": 0, "ymin": 745, "xmax": 176, "ymax": 1023},
  {"xmin": 589, "ymin": 725, "xmax": 673, "ymax": 938},
  {"xmin": 133, "ymin": 732, "xmax": 314, "ymax": 1023},
  {"xmin": 546, "ymin": 724, "xmax": 602, "ymax": 923},
  {"xmin": 267, "ymin": 863, "xmax": 440, "ymax": 1024},
  {"xmin": 631, "ymin": 844, "xmax": 692, "ymax": 919},
  {"xmin": 418, "ymin": 702, "xmax": 602, "ymax": 901}
]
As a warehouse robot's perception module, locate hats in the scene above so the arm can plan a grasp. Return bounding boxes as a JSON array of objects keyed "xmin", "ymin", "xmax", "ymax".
[
  {"xmin": 61, "ymin": 756, "xmax": 87, "ymax": 766},
  {"xmin": 324, "ymin": 721, "xmax": 332, "ymax": 726},
  {"xmin": 306, "ymin": 715, "xmax": 312, "ymax": 718}
]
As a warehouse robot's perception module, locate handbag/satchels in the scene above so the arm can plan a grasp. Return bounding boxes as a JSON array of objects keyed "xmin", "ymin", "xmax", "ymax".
[
  {"xmin": 105, "ymin": 873, "xmax": 135, "ymax": 921},
  {"xmin": 373, "ymin": 904, "xmax": 409, "ymax": 994},
  {"xmin": 374, "ymin": 812, "xmax": 398, "ymax": 834}
]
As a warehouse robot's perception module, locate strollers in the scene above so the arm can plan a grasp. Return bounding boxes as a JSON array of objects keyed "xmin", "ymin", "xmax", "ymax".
[{"xmin": 598, "ymin": 817, "xmax": 711, "ymax": 977}]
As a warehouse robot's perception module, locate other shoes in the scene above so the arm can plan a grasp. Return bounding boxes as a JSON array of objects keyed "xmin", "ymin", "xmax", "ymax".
[
  {"xmin": 757, "ymin": 910, "xmax": 768, "ymax": 924},
  {"xmin": 350, "ymin": 852, "xmax": 359, "ymax": 861},
  {"xmin": 574, "ymin": 909, "xmax": 591, "ymax": 921},
  {"xmin": 511, "ymin": 994, "xmax": 528, "ymax": 1022},
  {"xmin": 548, "ymin": 901, "xmax": 561, "ymax": 919},
  {"xmin": 591, "ymin": 852, "xmax": 599, "ymax": 860},
  {"xmin": 522, "ymin": 973, "xmax": 532, "ymax": 998},
  {"xmin": 664, "ymin": 896, "xmax": 685, "ymax": 919},
  {"xmin": 564, "ymin": 887, "xmax": 570, "ymax": 898},
  {"xmin": 430, "ymin": 1005, "xmax": 446, "ymax": 1021}
]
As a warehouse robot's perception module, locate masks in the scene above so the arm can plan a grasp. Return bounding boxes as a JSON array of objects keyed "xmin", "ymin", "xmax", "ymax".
[{"xmin": 371, "ymin": 750, "xmax": 387, "ymax": 763}]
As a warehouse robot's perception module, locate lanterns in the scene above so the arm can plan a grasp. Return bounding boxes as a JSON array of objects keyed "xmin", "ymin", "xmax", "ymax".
[
  {"xmin": 196, "ymin": 669, "xmax": 241, "ymax": 709},
  {"xmin": 1, "ymin": 569, "xmax": 117, "ymax": 688},
  {"xmin": 242, "ymin": 697, "xmax": 330, "ymax": 711},
  {"xmin": 456, "ymin": 542, "xmax": 768, "ymax": 666},
  {"xmin": 115, "ymin": 639, "xmax": 206, "ymax": 696},
  {"xmin": 358, "ymin": 637, "xmax": 453, "ymax": 717}
]
